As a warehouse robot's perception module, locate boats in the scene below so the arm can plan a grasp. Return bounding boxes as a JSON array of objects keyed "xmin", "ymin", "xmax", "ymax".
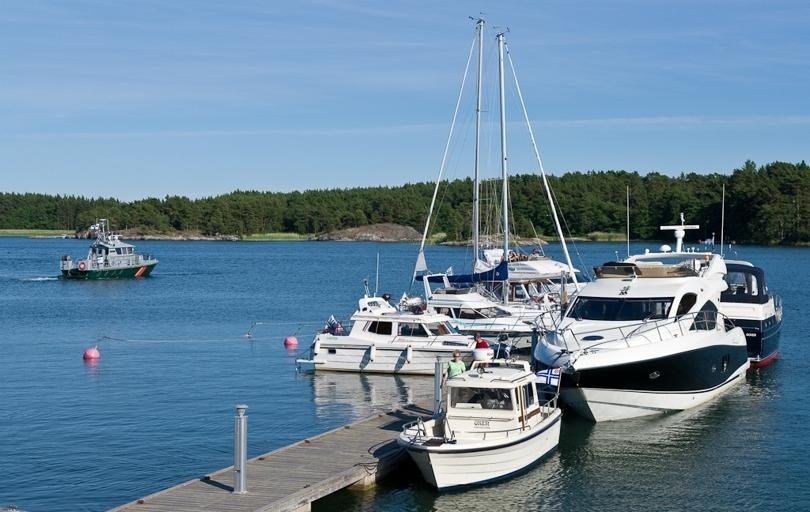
[
  {"xmin": 720, "ymin": 259, "xmax": 781, "ymax": 368},
  {"xmin": 297, "ymin": 252, "xmax": 513, "ymax": 373},
  {"xmin": 534, "ymin": 224, "xmax": 750, "ymax": 422},
  {"xmin": 399, "ymin": 358, "xmax": 563, "ymax": 489},
  {"xmin": 59, "ymin": 218, "xmax": 159, "ymax": 279}
]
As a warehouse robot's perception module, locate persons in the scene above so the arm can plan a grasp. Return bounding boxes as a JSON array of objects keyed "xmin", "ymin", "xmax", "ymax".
[
  {"xmin": 442, "ymin": 350, "xmax": 465, "ymax": 408},
  {"xmin": 474, "ymin": 332, "xmax": 492, "ymax": 368}
]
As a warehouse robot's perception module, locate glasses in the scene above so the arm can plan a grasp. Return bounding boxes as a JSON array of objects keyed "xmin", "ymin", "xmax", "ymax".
[{"xmin": 454, "ymin": 356, "xmax": 460, "ymax": 357}]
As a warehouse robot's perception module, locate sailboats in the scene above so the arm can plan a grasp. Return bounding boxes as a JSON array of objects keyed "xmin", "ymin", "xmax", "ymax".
[{"xmin": 394, "ymin": 19, "xmax": 592, "ymax": 354}]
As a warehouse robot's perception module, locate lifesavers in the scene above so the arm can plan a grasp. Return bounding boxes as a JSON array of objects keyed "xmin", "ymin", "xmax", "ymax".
[{"xmin": 79, "ymin": 262, "xmax": 85, "ymax": 269}]
